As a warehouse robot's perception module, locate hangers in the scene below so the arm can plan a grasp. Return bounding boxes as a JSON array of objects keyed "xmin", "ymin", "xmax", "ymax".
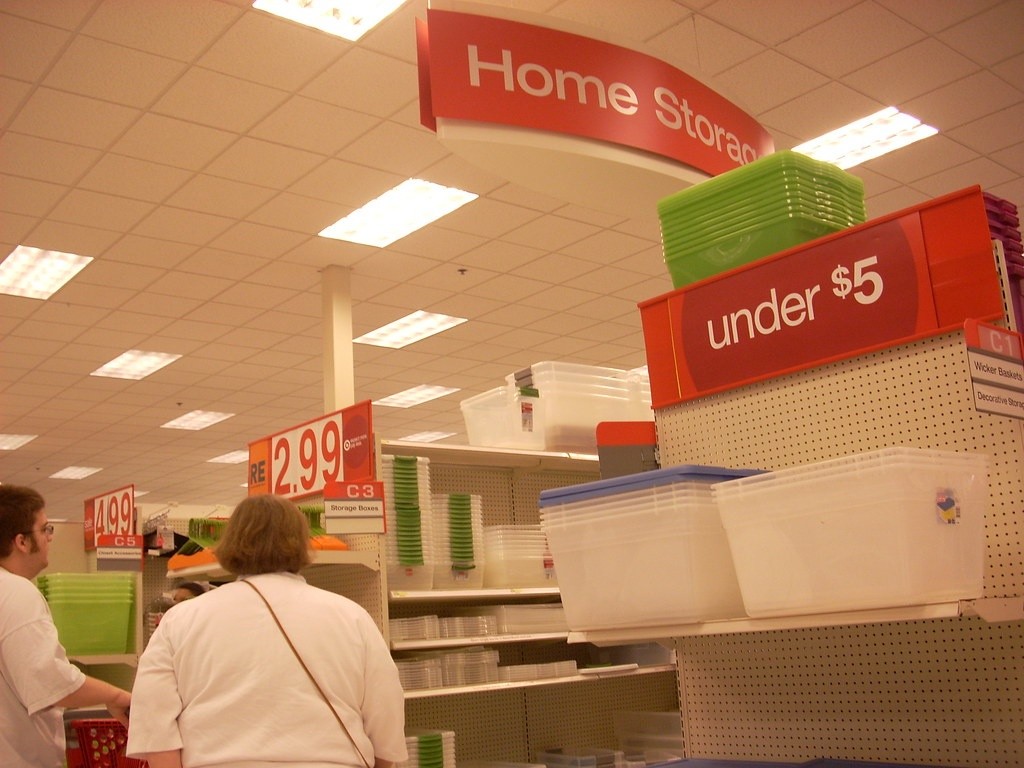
[
  {"xmin": 297, "ymin": 502, "xmax": 351, "ymax": 552},
  {"xmin": 144, "ymin": 508, "xmax": 230, "ymax": 573}
]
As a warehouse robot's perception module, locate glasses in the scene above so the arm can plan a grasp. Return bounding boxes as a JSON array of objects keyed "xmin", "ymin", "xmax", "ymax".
[{"xmin": 22, "ymin": 525, "xmax": 54, "ymax": 536}]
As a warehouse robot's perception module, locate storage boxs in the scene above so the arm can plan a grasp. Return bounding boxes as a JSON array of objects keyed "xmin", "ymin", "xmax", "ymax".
[
  {"xmin": 540, "ymin": 448, "xmax": 987, "ymax": 631},
  {"xmin": 656, "ymin": 149, "xmax": 869, "ymax": 290},
  {"xmin": 981, "ymin": 191, "xmax": 1023, "ymax": 334},
  {"xmin": 459, "ymin": 361, "xmax": 654, "ymax": 456},
  {"xmin": 379, "ymin": 454, "xmax": 558, "ymax": 589},
  {"xmin": 394, "ymin": 705, "xmax": 685, "ymax": 767},
  {"xmin": 36, "ymin": 570, "xmax": 136, "ymax": 658}
]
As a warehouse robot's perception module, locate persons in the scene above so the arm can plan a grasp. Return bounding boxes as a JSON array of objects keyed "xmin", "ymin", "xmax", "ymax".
[
  {"xmin": 126, "ymin": 493, "xmax": 408, "ymax": 768},
  {"xmin": 1, "ymin": 484, "xmax": 131, "ymax": 768}
]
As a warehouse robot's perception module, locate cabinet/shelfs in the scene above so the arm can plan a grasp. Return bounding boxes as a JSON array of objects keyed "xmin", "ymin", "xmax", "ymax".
[
  {"xmin": 294, "ymin": 432, "xmax": 679, "ymax": 768},
  {"xmin": 30, "ymin": 505, "xmax": 209, "ymax": 720},
  {"xmin": 633, "ymin": 238, "xmax": 1024, "ymax": 766}
]
